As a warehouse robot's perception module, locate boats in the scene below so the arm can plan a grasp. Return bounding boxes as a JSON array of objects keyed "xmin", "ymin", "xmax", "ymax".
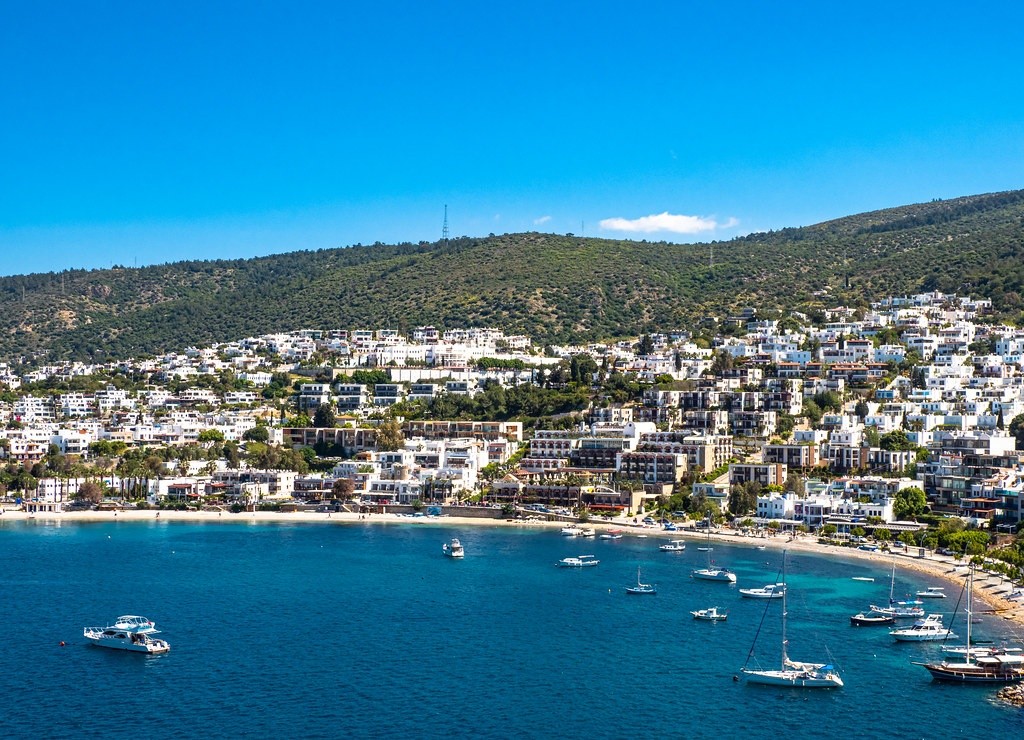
[
  {"xmin": 442, "ymin": 537, "xmax": 465, "ymax": 560},
  {"xmin": 599, "ymin": 529, "xmax": 623, "ymax": 540},
  {"xmin": 114, "ymin": 614, "xmax": 163, "ymax": 635},
  {"xmin": 689, "ymin": 608, "xmax": 728, "ymax": 621},
  {"xmin": 560, "ymin": 523, "xmax": 596, "ymax": 536},
  {"xmin": 738, "ymin": 582, "xmax": 788, "ymax": 599},
  {"xmin": 658, "ymin": 538, "xmax": 686, "ymax": 551},
  {"xmin": 637, "ymin": 535, "xmax": 648, "ymax": 539},
  {"xmin": 83, "ymin": 621, "xmax": 171, "ymax": 655},
  {"xmin": 557, "ymin": 555, "xmax": 601, "ymax": 566}
]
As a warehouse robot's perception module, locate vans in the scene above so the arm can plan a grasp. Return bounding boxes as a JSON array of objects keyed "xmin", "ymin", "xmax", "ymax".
[
  {"xmin": 525, "ymin": 504, "xmax": 545, "ymax": 511},
  {"xmin": 850, "ymin": 535, "xmax": 867, "ymax": 543}
]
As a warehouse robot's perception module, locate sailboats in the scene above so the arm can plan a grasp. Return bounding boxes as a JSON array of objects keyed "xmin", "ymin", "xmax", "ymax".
[
  {"xmin": 847, "ymin": 560, "xmax": 1024, "ymax": 684},
  {"xmin": 733, "ymin": 547, "xmax": 844, "ymax": 688},
  {"xmin": 625, "ymin": 566, "xmax": 658, "ymax": 594},
  {"xmin": 692, "ymin": 509, "xmax": 736, "ymax": 582}
]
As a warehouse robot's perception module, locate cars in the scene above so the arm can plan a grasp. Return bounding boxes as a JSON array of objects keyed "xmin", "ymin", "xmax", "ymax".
[
  {"xmin": 893, "ymin": 540, "xmax": 905, "ymax": 547},
  {"xmin": 859, "ymin": 544, "xmax": 877, "ymax": 551},
  {"xmin": 935, "ymin": 546, "xmax": 959, "ymax": 556}
]
